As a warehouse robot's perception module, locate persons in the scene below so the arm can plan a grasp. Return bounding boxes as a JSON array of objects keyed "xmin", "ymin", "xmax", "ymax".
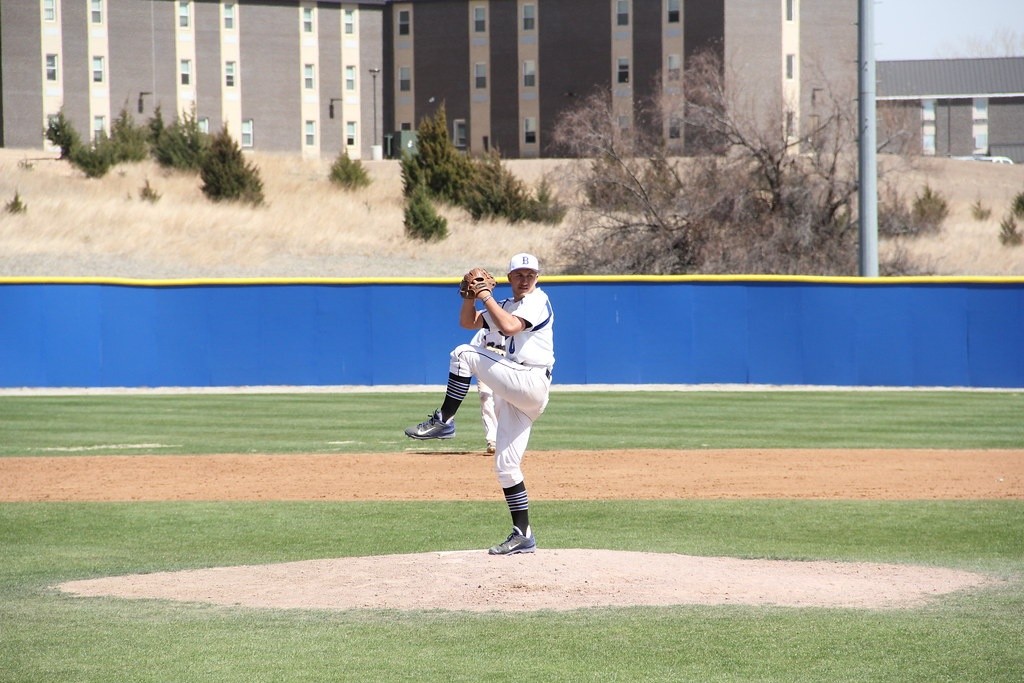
[
  {"xmin": 405, "ymin": 253, "xmax": 555, "ymax": 559},
  {"xmin": 470, "ymin": 328, "xmax": 506, "ymax": 454}
]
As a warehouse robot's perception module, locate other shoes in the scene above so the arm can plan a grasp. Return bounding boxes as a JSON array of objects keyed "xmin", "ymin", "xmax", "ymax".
[{"xmin": 486, "ymin": 442, "xmax": 496, "ymax": 453}]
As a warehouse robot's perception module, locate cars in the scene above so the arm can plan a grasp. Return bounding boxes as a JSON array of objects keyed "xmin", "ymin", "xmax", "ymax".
[{"xmin": 976, "ymin": 156, "xmax": 1015, "ymax": 165}]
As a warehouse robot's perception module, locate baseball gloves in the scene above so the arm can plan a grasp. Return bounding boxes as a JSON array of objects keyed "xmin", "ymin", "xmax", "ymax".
[{"xmin": 456, "ymin": 268, "xmax": 497, "ymax": 300}]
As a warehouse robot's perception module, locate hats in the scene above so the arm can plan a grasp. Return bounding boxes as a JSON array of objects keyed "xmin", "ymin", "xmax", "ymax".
[{"xmin": 506, "ymin": 253, "xmax": 539, "ymax": 274}]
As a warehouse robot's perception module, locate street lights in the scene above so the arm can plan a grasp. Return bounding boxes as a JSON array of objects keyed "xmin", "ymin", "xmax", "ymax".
[{"xmin": 368, "ymin": 68, "xmax": 382, "ymax": 146}]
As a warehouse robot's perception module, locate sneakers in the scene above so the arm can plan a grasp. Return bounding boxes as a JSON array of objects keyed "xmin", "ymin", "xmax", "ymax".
[
  {"xmin": 489, "ymin": 533, "xmax": 536, "ymax": 557},
  {"xmin": 404, "ymin": 408, "xmax": 455, "ymax": 439}
]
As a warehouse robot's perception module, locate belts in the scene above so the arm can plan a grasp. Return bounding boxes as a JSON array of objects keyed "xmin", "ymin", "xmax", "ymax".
[{"xmin": 545, "ymin": 370, "xmax": 550, "ymax": 378}]
